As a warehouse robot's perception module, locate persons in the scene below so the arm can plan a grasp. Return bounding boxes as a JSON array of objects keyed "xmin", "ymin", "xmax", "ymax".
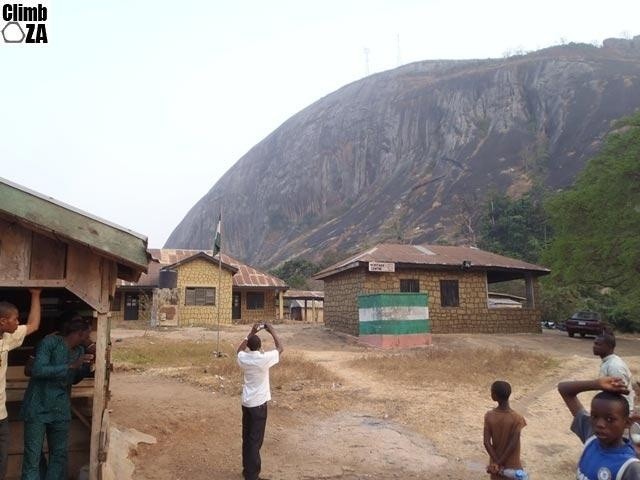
[
  {"xmin": 484, "ymin": 381, "xmax": 527, "ymax": 480},
  {"xmin": 237, "ymin": 323, "xmax": 283, "ymax": 480},
  {"xmin": 558, "ymin": 377, "xmax": 640, "ymax": 480},
  {"xmin": 593, "ymin": 335, "xmax": 634, "ymax": 411},
  {"xmin": 0, "ymin": 287, "xmax": 96, "ymax": 480}
]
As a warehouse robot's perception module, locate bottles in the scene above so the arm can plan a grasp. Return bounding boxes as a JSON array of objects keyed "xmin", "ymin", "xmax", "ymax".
[{"xmin": 497, "ymin": 467, "xmax": 529, "ymax": 480}]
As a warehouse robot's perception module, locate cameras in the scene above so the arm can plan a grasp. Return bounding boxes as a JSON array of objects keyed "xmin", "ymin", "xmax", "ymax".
[{"xmin": 259, "ymin": 324, "xmax": 266, "ymax": 329}]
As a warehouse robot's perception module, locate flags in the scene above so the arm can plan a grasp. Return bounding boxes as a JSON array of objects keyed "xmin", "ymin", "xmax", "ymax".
[{"xmin": 213, "ymin": 213, "xmax": 221, "ymax": 257}]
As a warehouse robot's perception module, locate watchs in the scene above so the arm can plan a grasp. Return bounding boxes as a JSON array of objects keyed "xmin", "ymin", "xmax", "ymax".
[{"xmin": 244, "ymin": 337, "xmax": 248, "ymax": 340}]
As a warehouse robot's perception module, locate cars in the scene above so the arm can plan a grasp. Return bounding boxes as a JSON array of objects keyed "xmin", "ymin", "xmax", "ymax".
[
  {"xmin": 540, "ymin": 317, "xmax": 567, "ymax": 331},
  {"xmin": 564, "ymin": 310, "xmax": 615, "ymax": 339}
]
